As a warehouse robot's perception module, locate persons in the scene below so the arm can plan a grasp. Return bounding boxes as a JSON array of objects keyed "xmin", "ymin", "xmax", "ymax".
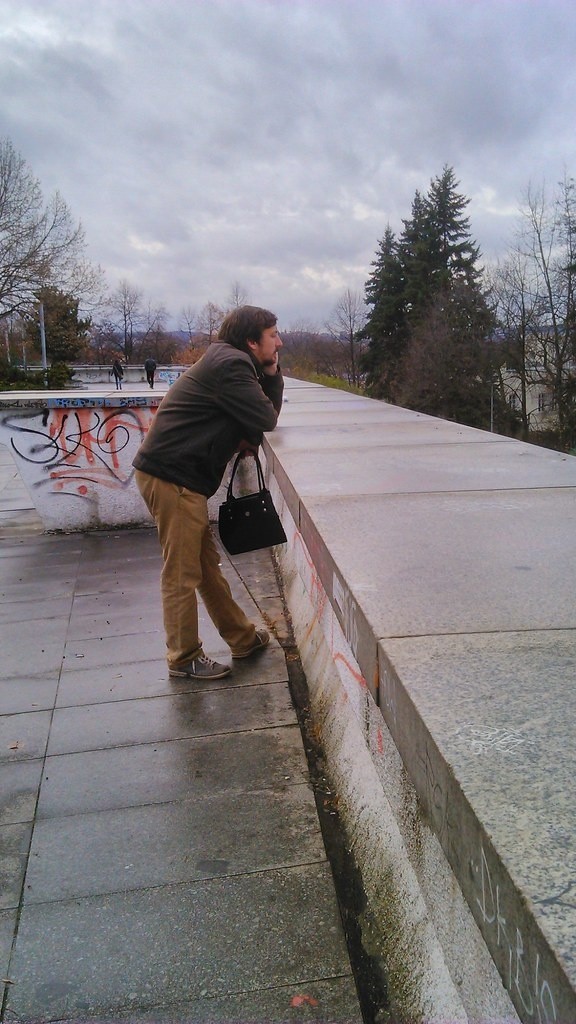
[
  {"xmin": 111, "ymin": 360, "xmax": 123, "ymax": 389},
  {"xmin": 132, "ymin": 305, "xmax": 285, "ymax": 679},
  {"xmin": 145, "ymin": 355, "xmax": 156, "ymax": 389}
]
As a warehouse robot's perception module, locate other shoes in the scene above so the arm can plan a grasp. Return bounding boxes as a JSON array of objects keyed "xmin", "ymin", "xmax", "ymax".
[
  {"xmin": 229, "ymin": 629, "xmax": 270, "ymax": 658},
  {"xmin": 168, "ymin": 655, "xmax": 232, "ymax": 680}
]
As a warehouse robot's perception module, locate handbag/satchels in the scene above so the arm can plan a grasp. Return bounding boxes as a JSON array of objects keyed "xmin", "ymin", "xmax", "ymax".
[
  {"xmin": 219, "ymin": 448, "xmax": 288, "ymax": 555},
  {"xmin": 118, "ymin": 372, "xmax": 124, "ymax": 379}
]
